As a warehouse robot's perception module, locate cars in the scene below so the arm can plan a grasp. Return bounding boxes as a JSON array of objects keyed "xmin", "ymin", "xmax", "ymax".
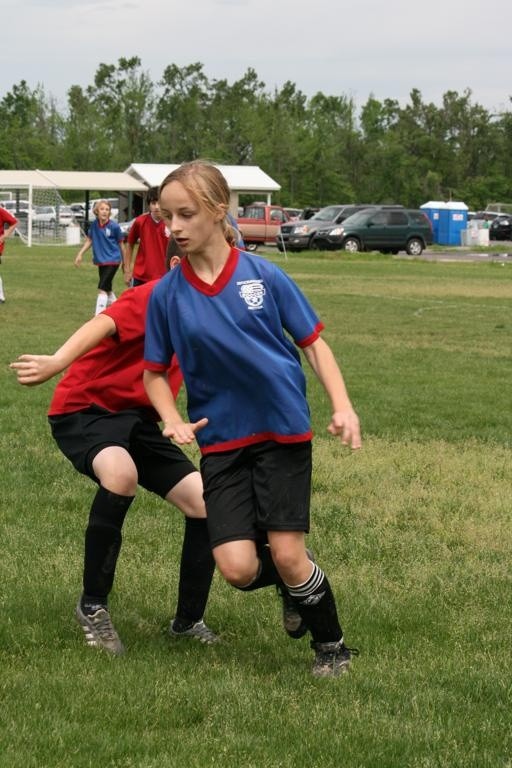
[
  {"xmin": 281, "ymin": 205, "xmax": 324, "ymax": 223},
  {"xmin": 0, "ymin": 198, "xmax": 119, "ymax": 233},
  {"xmin": 466, "ymin": 211, "xmax": 511, "ymax": 240}
]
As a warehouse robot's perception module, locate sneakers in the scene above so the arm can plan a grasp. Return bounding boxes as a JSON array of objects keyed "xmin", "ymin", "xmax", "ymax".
[
  {"xmin": 167, "ymin": 620, "xmax": 223, "ymax": 647},
  {"xmin": 279, "ymin": 548, "xmax": 315, "ymax": 639},
  {"xmin": 75, "ymin": 603, "xmax": 124, "ymax": 653},
  {"xmin": 309, "ymin": 637, "xmax": 350, "ymax": 677}
]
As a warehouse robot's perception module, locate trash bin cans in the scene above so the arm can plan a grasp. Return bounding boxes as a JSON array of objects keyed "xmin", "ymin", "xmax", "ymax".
[
  {"xmin": 65, "ymin": 228, "xmax": 80, "ymax": 246},
  {"xmin": 14, "ymin": 212, "xmax": 28, "ymax": 236},
  {"xmin": 419, "ymin": 201, "xmax": 469, "ymax": 245}
]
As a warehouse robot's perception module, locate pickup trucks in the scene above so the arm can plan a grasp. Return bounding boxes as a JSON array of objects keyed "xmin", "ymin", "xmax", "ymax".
[{"xmin": 230, "ymin": 205, "xmax": 293, "ymax": 252}]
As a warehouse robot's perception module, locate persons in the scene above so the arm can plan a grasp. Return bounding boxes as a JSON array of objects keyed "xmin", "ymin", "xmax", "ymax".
[
  {"xmin": 72, "ymin": 195, "xmax": 127, "ymax": 316},
  {"xmin": 8, "ymin": 222, "xmax": 234, "ymax": 656},
  {"xmin": 0, "ymin": 203, "xmax": 21, "ymax": 305},
  {"xmin": 124, "ymin": 184, "xmax": 172, "ymax": 288},
  {"xmin": 223, "ymin": 210, "xmax": 248, "ymax": 252},
  {"xmin": 140, "ymin": 158, "xmax": 367, "ymax": 683}
]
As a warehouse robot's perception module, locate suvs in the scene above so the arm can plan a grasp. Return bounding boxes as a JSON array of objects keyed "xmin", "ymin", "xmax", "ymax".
[
  {"xmin": 314, "ymin": 207, "xmax": 433, "ymax": 256},
  {"xmin": 276, "ymin": 204, "xmax": 405, "ymax": 253}
]
